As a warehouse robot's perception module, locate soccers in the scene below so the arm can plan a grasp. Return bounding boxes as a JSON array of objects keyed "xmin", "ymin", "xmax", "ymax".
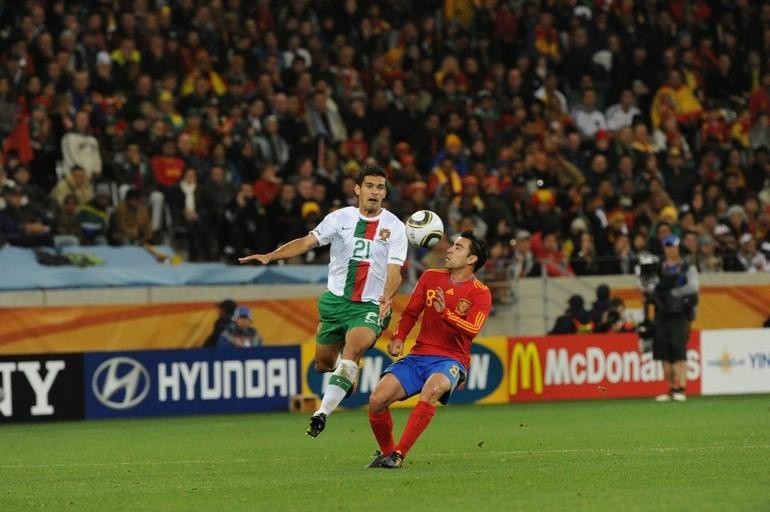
[{"xmin": 406, "ymin": 209, "xmax": 444, "ymax": 249}]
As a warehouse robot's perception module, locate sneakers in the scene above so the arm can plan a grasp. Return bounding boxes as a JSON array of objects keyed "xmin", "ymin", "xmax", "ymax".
[
  {"xmin": 366, "ymin": 454, "xmax": 385, "ymax": 468},
  {"xmin": 305, "ymin": 412, "xmax": 327, "ymax": 440},
  {"xmin": 379, "ymin": 450, "xmax": 404, "ymax": 469},
  {"xmin": 654, "ymin": 389, "xmax": 689, "ymax": 403}
]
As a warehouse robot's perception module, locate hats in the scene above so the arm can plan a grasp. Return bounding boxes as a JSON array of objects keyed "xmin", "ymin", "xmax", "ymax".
[
  {"xmin": 233, "ymin": 307, "xmax": 252, "ymax": 319},
  {"xmin": 661, "ymin": 234, "xmax": 682, "ymax": 248}
]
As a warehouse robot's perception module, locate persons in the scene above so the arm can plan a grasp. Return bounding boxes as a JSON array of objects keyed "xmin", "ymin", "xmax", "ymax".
[
  {"xmin": 638, "ymin": 234, "xmax": 699, "ymax": 402},
  {"xmin": 365, "ymin": 230, "xmax": 492, "ymax": 469},
  {"xmin": 237, "ymin": 165, "xmax": 409, "ymax": 438}
]
